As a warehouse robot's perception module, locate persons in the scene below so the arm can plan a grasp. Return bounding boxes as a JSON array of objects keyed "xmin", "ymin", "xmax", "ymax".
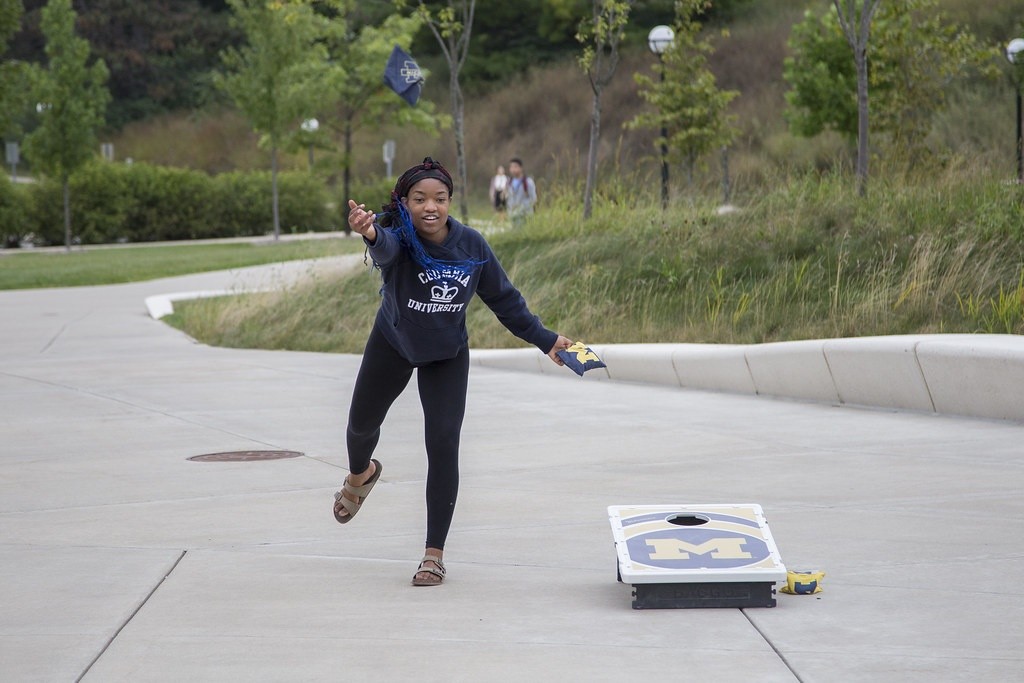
[
  {"xmin": 332, "ymin": 156, "xmax": 572, "ymax": 585},
  {"xmin": 488, "ymin": 165, "xmax": 509, "ymax": 208},
  {"xmin": 506, "ymin": 158, "xmax": 537, "ymax": 228}
]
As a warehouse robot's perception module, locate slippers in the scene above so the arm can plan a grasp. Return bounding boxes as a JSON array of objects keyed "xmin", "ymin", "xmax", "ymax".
[
  {"xmin": 411, "ymin": 555, "xmax": 447, "ymax": 586},
  {"xmin": 333, "ymin": 458, "xmax": 382, "ymax": 524}
]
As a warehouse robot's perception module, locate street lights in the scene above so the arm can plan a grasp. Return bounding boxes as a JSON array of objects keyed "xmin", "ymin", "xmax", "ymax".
[
  {"xmin": 648, "ymin": 25, "xmax": 675, "ymax": 211},
  {"xmin": 1006, "ymin": 38, "xmax": 1024, "ymax": 180}
]
